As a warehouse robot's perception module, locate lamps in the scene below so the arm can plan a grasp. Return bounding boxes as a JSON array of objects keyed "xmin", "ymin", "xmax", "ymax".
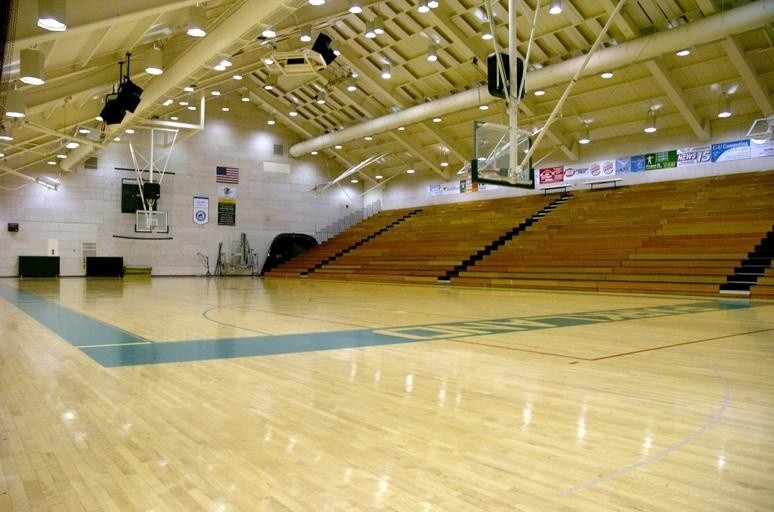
[
  {"xmin": 480, "ymin": 22, "xmax": 492, "ymax": 41},
  {"xmin": 300, "ymin": 24, "xmax": 312, "ymax": 43},
  {"xmin": 380, "ymin": 63, "xmax": 391, "ymax": 79},
  {"xmin": 426, "ymin": 44, "xmax": 438, "ymax": 61},
  {"xmin": 364, "ymin": 4, "xmax": 385, "ymax": 39},
  {"xmin": 5, "ymin": 0, "xmax": 68, "ymax": 120},
  {"xmin": 98, "ymin": 5, "xmax": 207, "ymax": 127}
]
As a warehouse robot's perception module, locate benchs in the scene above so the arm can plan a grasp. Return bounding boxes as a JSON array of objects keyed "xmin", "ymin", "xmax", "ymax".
[{"xmin": 262, "ymin": 167, "xmax": 774, "ymax": 300}]
